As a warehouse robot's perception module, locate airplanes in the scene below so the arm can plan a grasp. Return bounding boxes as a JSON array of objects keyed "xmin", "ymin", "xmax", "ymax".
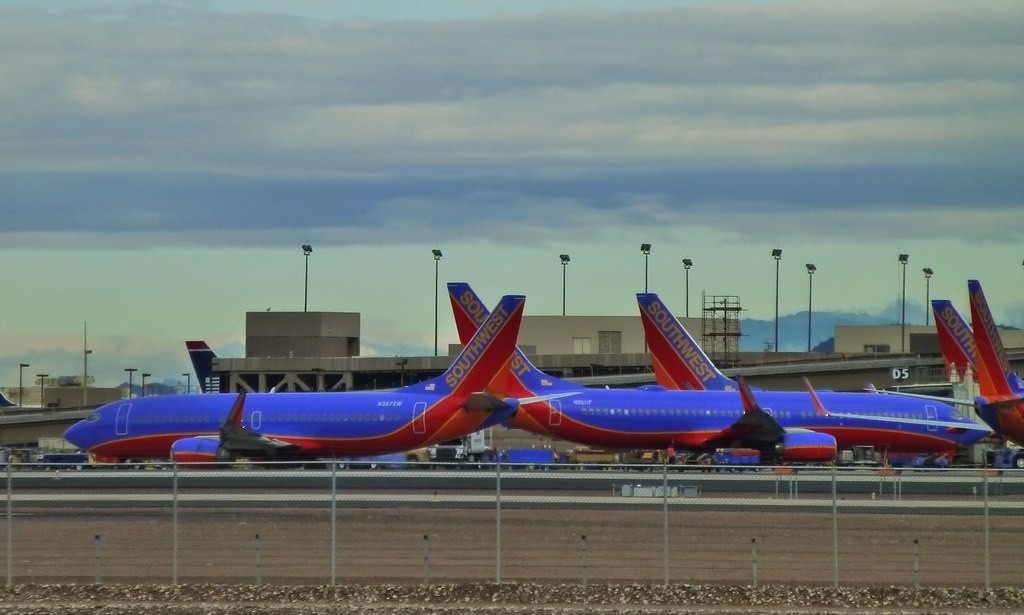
[
  {"xmin": 930, "ymin": 278, "xmax": 1024, "ymax": 471},
  {"xmin": 443, "ymin": 279, "xmax": 997, "ymax": 472},
  {"xmin": 59, "ymin": 293, "xmax": 584, "ymax": 471}
]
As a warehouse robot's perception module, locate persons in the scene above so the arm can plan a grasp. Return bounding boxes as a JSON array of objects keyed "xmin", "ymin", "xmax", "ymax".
[{"xmin": 667, "ymin": 444, "xmax": 675, "ymax": 470}]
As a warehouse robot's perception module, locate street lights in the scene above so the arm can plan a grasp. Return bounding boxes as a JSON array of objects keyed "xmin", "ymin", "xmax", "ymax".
[
  {"xmin": 682, "ymin": 257, "xmax": 693, "ymax": 317},
  {"xmin": 19, "ymin": 362, "xmax": 30, "ymax": 407},
  {"xmin": 639, "ymin": 243, "xmax": 652, "ymax": 353},
  {"xmin": 311, "ymin": 367, "xmax": 325, "ymax": 392},
  {"xmin": 921, "ymin": 266, "xmax": 935, "ymax": 327},
  {"xmin": 394, "ymin": 359, "xmax": 409, "ymax": 388},
  {"xmin": 301, "ymin": 245, "xmax": 314, "ymax": 311},
  {"xmin": 559, "ymin": 254, "xmax": 571, "ymax": 316},
  {"xmin": 897, "ymin": 253, "xmax": 912, "ymax": 351},
  {"xmin": 771, "ymin": 248, "xmax": 783, "ymax": 352},
  {"xmin": 431, "ymin": 248, "xmax": 443, "ymax": 356},
  {"xmin": 124, "ymin": 368, "xmax": 137, "ymax": 399},
  {"xmin": 141, "ymin": 373, "xmax": 152, "ymax": 397},
  {"xmin": 182, "ymin": 373, "xmax": 190, "ymax": 394},
  {"xmin": 805, "ymin": 263, "xmax": 818, "ymax": 353},
  {"xmin": 35, "ymin": 373, "xmax": 50, "ymax": 407}
]
